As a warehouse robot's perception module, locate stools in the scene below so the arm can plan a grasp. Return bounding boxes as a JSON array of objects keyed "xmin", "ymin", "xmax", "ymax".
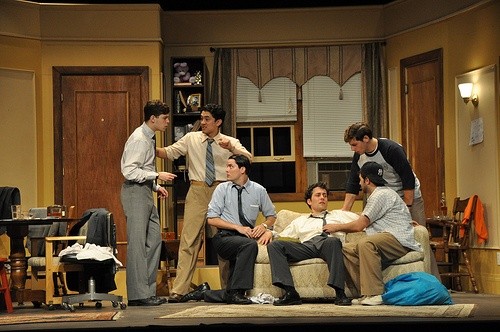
[{"xmin": 61, "ymin": 249, "xmax": 128, "ymax": 313}]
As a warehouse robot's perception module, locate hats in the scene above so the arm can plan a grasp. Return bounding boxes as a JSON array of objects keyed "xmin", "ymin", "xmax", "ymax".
[{"xmin": 360, "ymin": 161, "xmax": 388, "ymax": 185}]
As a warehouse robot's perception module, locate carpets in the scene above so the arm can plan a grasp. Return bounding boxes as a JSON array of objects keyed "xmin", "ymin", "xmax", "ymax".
[
  {"xmin": 154, "ymin": 297, "xmax": 479, "ymax": 319},
  {"xmin": 0, "ymin": 311, "xmax": 122, "ymax": 323}
]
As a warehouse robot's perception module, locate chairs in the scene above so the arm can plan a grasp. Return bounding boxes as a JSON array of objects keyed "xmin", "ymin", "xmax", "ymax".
[
  {"xmin": 426, "ymin": 193, "xmax": 482, "ymax": 294},
  {"xmin": 29, "ymin": 206, "xmax": 116, "ymax": 308}
]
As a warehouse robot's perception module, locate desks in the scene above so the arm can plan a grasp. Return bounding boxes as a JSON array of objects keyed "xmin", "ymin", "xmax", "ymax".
[{"xmin": 0, "ymin": 216, "xmax": 85, "ymax": 309}]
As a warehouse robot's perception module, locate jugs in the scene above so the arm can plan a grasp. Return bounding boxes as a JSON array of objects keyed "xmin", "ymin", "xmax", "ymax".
[{"xmin": 47, "ymin": 202, "xmax": 67, "ymax": 219}]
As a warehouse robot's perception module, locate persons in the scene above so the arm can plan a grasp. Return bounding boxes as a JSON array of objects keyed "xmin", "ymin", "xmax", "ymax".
[
  {"xmin": 182, "ymin": 153, "xmax": 277, "ymax": 304},
  {"xmin": 258, "ymin": 183, "xmax": 419, "ymax": 306},
  {"xmin": 156, "ymin": 104, "xmax": 252, "ymax": 303},
  {"xmin": 119, "ymin": 100, "xmax": 178, "ymax": 306},
  {"xmin": 322, "ymin": 162, "xmax": 422, "ymax": 305},
  {"xmin": 342, "ymin": 122, "xmax": 441, "ymax": 282}
]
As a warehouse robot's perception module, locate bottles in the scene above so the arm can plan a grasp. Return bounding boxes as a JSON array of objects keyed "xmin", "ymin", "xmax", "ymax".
[{"xmin": 440, "ymin": 193, "xmax": 446, "ymax": 207}]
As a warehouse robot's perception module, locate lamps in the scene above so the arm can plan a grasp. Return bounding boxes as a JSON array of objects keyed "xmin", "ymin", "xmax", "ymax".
[{"xmin": 457, "ymin": 82, "xmax": 479, "ymax": 109}]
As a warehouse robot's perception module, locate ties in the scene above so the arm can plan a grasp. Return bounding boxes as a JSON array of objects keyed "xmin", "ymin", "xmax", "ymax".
[
  {"xmin": 205, "ymin": 138, "xmax": 214, "ymax": 186},
  {"xmin": 235, "ymin": 185, "xmax": 254, "ymax": 230},
  {"xmin": 153, "ymin": 135, "xmax": 159, "ymax": 186},
  {"xmin": 310, "ymin": 211, "xmax": 330, "ymax": 236}
]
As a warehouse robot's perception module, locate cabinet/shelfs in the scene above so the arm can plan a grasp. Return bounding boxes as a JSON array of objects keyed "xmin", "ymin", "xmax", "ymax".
[{"xmin": 169, "ymin": 52, "xmax": 213, "ymax": 239}]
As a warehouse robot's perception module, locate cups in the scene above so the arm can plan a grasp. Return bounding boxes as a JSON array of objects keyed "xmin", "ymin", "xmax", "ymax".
[{"xmin": 11, "ymin": 205, "xmax": 28, "ymax": 219}]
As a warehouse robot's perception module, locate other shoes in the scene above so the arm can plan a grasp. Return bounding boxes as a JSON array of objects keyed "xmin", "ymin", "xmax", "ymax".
[
  {"xmin": 168, "ymin": 292, "xmax": 183, "ymax": 303},
  {"xmin": 227, "ymin": 292, "xmax": 251, "ymax": 304},
  {"xmin": 180, "ymin": 282, "xmax": 210, "ymax": 303},
  {"xmin": 272, "ymin": 291, "xmax": 302, "ymax": 307},
  {"xmin": 128, "ymin": 296, "xmax": 167, "ymax": 306},
  {"xmin": 361, "ymin": 295, "xmax": 382, "ymax": 305},
  {"xmin": 350, "ymin": 296, "xmax": 367, "ymax": 304},
  {"xmin": 334, "ymin": 295, "xmax": 352, "ymax": 306}
]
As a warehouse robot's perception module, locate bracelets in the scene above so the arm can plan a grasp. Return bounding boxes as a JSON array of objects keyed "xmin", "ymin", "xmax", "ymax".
[
  {"xmin": 407, "ymin": 205, "xmax": 412, "ymax": 207},
  {"xmin": 262, "ymin": 223, "xmax": 267, "ymax": 228}
]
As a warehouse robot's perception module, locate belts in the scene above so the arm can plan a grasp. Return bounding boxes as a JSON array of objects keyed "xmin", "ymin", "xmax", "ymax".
[{"xmin": 190, "ymin": 180, "xmax": 221, "ymax": 186}]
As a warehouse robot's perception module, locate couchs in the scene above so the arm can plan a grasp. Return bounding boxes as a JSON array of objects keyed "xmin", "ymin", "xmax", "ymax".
[{"xmin": 247, "ymin": 206, "xmax": 433, "ymax": 300}]
